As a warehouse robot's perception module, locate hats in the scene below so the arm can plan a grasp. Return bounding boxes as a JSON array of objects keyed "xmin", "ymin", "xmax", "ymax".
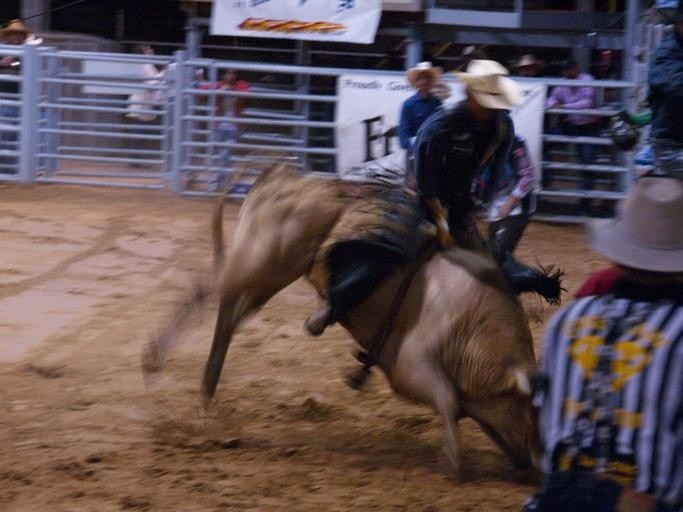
[
  {"xmin": 515, "ymin": 54, "xmax": 541, "ymax": 66},
  {"xmin": 406, "ymin": 61, "xmax": 443, "ymax": 88},
  {"xmin": 0, "ymin": 19, "xmax": 33, "ymax": 34},
  {"xmin": 593, "ymin": 176, "xmax": 683, "ymax": 274},
  {"xmin": 451, "ymin": 59, "xmax": 523, "ymax": 113}
]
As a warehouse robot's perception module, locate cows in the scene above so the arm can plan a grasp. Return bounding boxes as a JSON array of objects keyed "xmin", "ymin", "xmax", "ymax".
[{"xmin": 140, "ymin": 147, "xmax": 543, "ymax": 486}]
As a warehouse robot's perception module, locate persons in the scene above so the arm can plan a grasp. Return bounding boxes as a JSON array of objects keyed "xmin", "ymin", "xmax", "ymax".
[
  {"xmin": 541, "ymin": 1, "xmax": 683, "ymax": 212},
  {"xmin": 0, "ymin": 18, "xmax": 31, "ymax": 174},
  {"xmin": 303, "ymin": 57, "xmax": 536, "ymax": 337},
  {"xmin": 535, "ymin": 176, "xmax": 683, "ymax": 511},
  {"xmin": 127, "ymin": 43, "xmax": 251, "ymax": 189}
]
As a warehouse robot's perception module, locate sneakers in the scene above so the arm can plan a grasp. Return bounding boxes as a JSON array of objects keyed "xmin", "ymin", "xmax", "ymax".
[{"xmin": 305, "ymin": 309, "xmax": 327, "ymax": 336}]
